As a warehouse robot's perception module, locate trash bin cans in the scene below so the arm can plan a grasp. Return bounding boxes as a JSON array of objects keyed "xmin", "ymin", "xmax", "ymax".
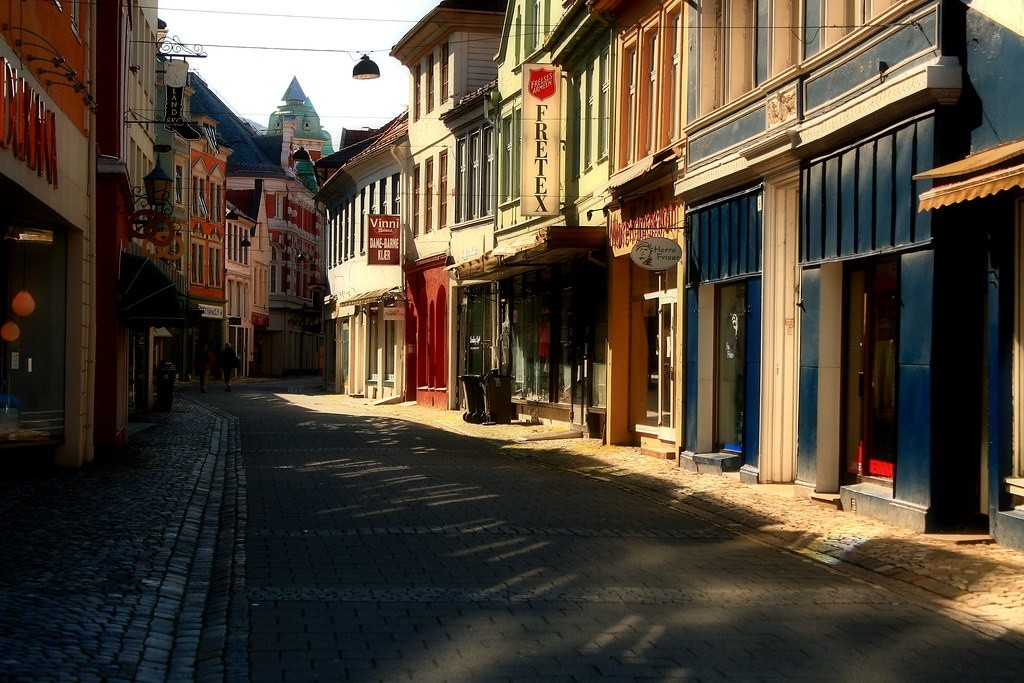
[
  {"xmin": 456, "ymin": 374, "xmax": 487, "ymax": 424},
  {"xmin": 480, "ymin": 373, "xmax": 514, "ymax": 425}
]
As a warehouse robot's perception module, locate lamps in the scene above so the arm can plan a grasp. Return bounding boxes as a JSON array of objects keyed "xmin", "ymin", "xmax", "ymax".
[
  {"xmin": 4, "ymin": 23, "xmax": 101, "ymax": 114},
  {"xmin": 143, "ymin": 154, "xmax": 175, "ymax": 207},
  {"xmin": 241, "ymin": 236, "xmax": 251, "ymax": 247},
  {"xmin": 351, "ymin": 51, "xmax": 381, "ymax": 81},
  {"xmin": 225, "ymin": 208, "xmax": 239, "ymax": 221}
]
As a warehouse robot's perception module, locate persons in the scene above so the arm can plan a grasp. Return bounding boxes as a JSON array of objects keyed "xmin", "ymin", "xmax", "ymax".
[
  {"xmin": 219, "ymin": 343, "xmax": 240, "ymax": 392},
  {"xmin": 195, "ymin": 342, "xmax": 215, "ymax": 394}
]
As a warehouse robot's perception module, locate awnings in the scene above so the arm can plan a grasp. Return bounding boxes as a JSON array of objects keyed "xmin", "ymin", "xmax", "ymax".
[{"xmin": 116, "ymin": 252, "xmax": 185, "ymax": 329}]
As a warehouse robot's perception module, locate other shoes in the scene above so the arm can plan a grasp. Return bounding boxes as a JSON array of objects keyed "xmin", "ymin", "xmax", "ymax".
[
  {"xmin": 224, "ymin": 386, "xmax": 232, "ymax": 392},
  {"xmin": 200, "ymin": 386, "xmax": 208, "ymax": 393}
]
